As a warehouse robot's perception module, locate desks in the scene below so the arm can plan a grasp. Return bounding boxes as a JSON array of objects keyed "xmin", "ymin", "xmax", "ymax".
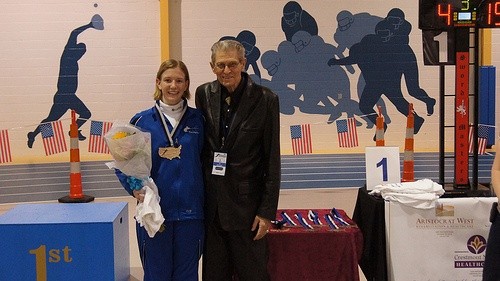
[
  {"xmin": 268, "ymin": 209, "xmax": 364, "ymax": 281},
  {"xmin": 351, "ymin": 185, "xmax": 500, "ymax": 281}
]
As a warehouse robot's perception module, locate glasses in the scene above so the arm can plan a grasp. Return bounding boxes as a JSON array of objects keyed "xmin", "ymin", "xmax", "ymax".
[{"xmin": 214, "ymin": 61, "xmax": 241, "ymax": 70}]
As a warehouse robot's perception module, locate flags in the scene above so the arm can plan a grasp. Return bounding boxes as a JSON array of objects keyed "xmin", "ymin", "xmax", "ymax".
[
  {"xmin": 88, "ymin": 121, "xmax": 113, "ymax": 154},
  {"xmin": 0, "ymin": 129, "xmax": 12, "ymax": 163},
  {"xmin": 289, "ymin": 123, "xmax": 312, "ymax": 155},
  {"xmin": 336, "ymin": 117, "xmax": 358, "ymax": 147},
  {"xmin": 40, "ymin": 120, "xmax": 67, "ymax": 156},
  {"xmin": 469, "ymin": 124, "xmax": 489, "ymax": 155}
]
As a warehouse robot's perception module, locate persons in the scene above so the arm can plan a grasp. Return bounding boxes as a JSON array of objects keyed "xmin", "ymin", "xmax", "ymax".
[
  {"xmin": 195, "ymin": 40, "xmax": 281, "ymax": 281},
  {"xmin": 115, "ymin": 59, "xmax": 207, "ymax": 281}
]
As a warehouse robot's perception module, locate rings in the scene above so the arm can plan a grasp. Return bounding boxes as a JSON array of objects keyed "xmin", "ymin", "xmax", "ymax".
[{"xmin": 266, "ymin": 230, "xmax": 269, "ymax": 233}]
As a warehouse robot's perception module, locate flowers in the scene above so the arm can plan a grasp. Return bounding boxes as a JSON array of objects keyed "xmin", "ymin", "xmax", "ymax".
[{"xmin": 110, "ymin": 131, "xmax": 166, "ymax": 233}]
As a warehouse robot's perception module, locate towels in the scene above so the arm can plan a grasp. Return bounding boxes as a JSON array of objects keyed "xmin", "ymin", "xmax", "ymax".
[
  {"xmin": 136, "ymin": 186, "xmax": 165, "ymax": 237},
  {"xmin": 368, "ymin": 178, "xmax": 445, "ymax": 209}
]
